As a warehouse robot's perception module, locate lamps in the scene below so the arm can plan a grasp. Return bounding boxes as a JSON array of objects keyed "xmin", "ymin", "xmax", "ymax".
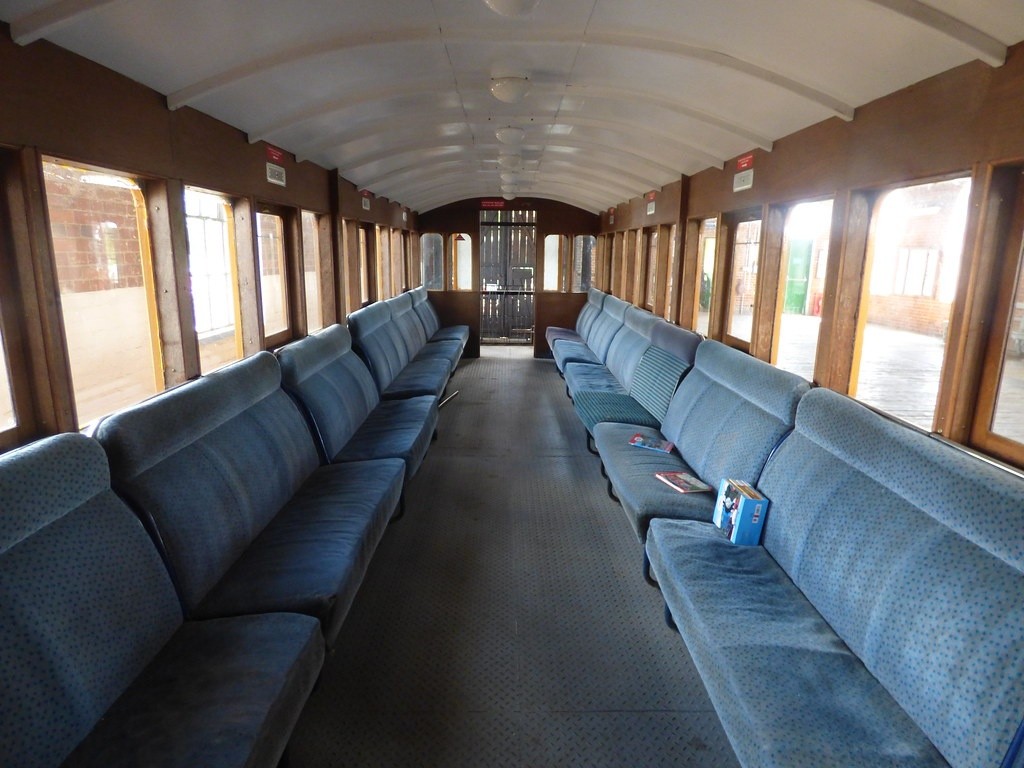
[
  {"xmin": 501, "ymin": 173, "xmax": 519, "ymax": 182},
  {"xmin": 455, "ymin": 233, "xmax": 465, "ymax": 240},
  {"xmin": 502, "ymin": 194, "xmax": 515, "ymax": 200},
  {"xmin": 489, "ymin": 76, "xmax": 532, "ymax": 103},
  {"xmin": 497, "ymin": 154, "xmax": 521, "ymax": 169},
  {"xmin": 496, "ymin": 127, "xmax": 525, "ymax": 145},
  {"xmin": 484, "ymin": 0, "xmax": 541, "ymax": 17},
  {"xmin": 501, "ymin": 183, "xmax": 518, "ymax": 192}
]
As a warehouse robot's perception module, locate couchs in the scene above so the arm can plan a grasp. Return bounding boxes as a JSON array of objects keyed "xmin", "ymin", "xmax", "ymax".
[
  {"xmin": 385, "ymin": 292, "xmax": 464, "ymax": 373},
  {"xmin": 643, "ymin": 385, "xmax": 1024, "ymax": 768},
  {"xmin": 90, "ymin": 351, "xmax": 406, "ymax": 655},
  {"xmin": 593, "ymin": 336, "xmax": 817, "ymax": 548},
  {"xmin": 346, "ymin": 301, "xmax": 451, "ymax": 401},
  {"xmin": 544, "ymin": 287, "xmax": 610, "ymax": 361},
  {"xmin": 409, "ymin": 284, "xmax": 470, "ymax": 348},
  {"xmin": 553, "ymin": 294, "xmax": 634, "ymax": 381},
  {"xmin": 274, "ymin": 323, "xmax": 440, "ymax": 486},
  {"xmin": 0, "ymin": 432, "xmax": 330, "ymax": 768},
  {"xmin": 563, "ymin": 304, "xmax": 668, "ymax": 405},
  {"xmin": 575, "ymin": 321, "xmax": 707, "ymax": 455}
]
